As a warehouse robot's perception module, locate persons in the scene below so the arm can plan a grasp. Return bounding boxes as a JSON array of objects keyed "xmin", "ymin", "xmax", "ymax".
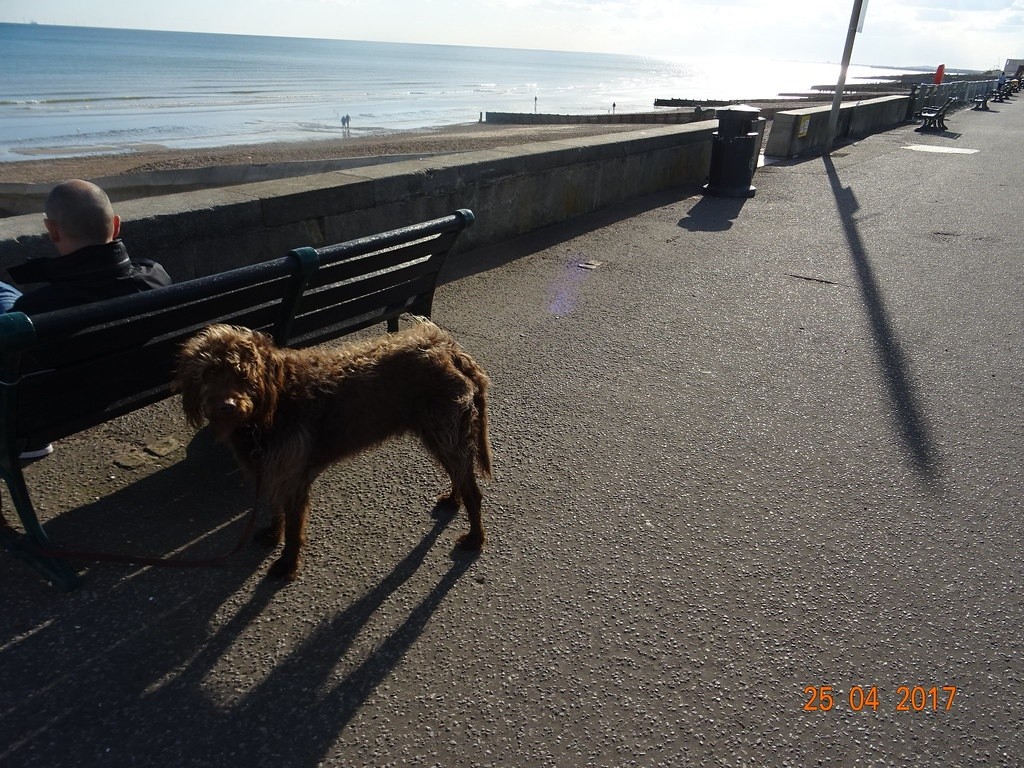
[
  {"xmin": 0, "ymin": 180, "xmax": 171, "ymax": 459},
  {"xmin": 1017, "ymin": 73, "xmax": 1022, "ymax": 91},
  {"xmin": 997, "ymin": 72, "xmax": 1005, "ymax": 90}
]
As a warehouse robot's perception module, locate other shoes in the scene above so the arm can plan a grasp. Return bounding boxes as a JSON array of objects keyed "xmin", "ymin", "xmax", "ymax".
[{"xmin": 19, "ymin": 444, "xmax": 53, "ymax": 458}]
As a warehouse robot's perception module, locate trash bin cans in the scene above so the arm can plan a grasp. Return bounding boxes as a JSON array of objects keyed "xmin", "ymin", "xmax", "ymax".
[{"xmin": 702, "ymin": 104, "xmax": 761, "ymax": 197}]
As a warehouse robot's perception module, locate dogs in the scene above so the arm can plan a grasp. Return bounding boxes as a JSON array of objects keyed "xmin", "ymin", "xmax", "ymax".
[{"xmin": 174, "ymin": 323, "xmax": 493, "ymax": 583}]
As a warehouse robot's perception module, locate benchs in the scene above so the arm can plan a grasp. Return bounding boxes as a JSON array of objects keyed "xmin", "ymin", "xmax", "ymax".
[
  {"xmin": 913, "ymin": 96, "xmax": 959, "ymax": 132},
  {"xmin": 968, "ymin": 82, "xmax": 1024, "ymax": 111},
  {"xmin": 0, "ymin": 209, "xmax": 475, "ymax": 588}
]
